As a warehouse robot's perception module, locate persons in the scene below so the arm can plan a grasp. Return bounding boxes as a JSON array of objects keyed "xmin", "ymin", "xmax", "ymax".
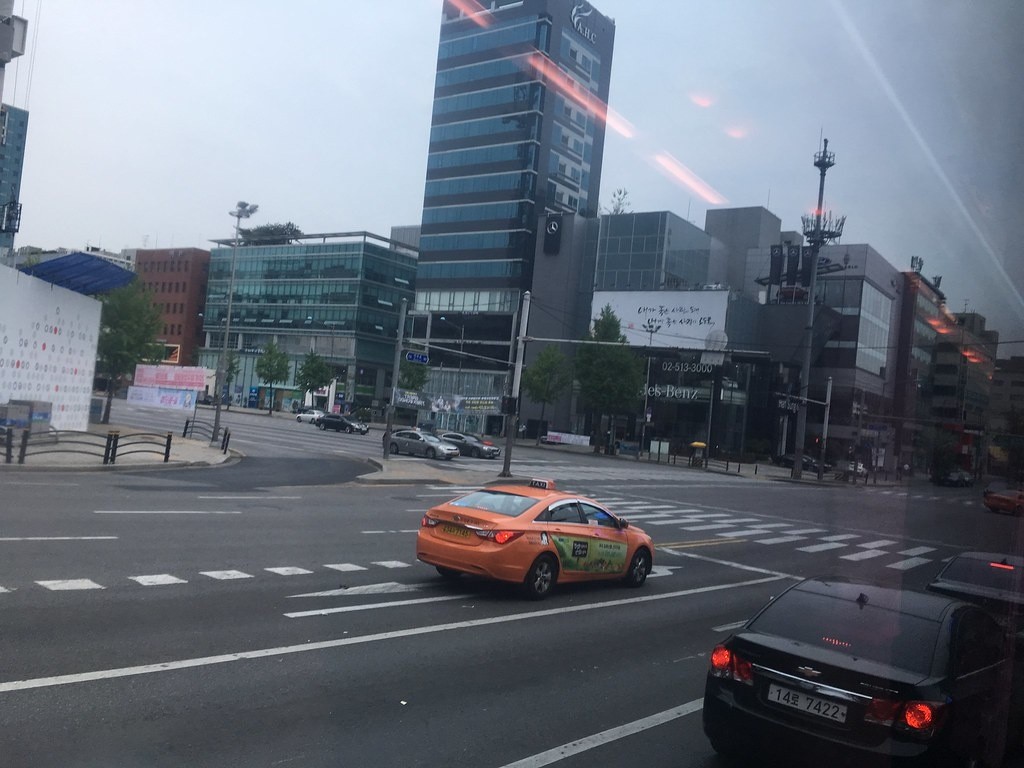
[
  {"xmin": 432, "ymin": 395, "xmax": 451, "ymax": 412},
  {"xmin": 411, "ymin": 425, "xmax": 420, "ymax": 431}
]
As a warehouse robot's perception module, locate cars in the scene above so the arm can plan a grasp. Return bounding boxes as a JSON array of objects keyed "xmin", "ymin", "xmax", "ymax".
[
  {"xmin": 962, "ymin": 473, "xmax": 973, "ymax": 482},
  {"xmin": 541, "ymin": 430, "xmax": 574, "ymax": 445},
  {"xmin": 298, "ymin": 406, "xmax": 312, "ymax": 414},
  {"xmin": 926, "ymin": 551, "xmax": 1024, "ymax": 644},
  {"xmin": 782, "ymin": 453, "xmax": 831, "ymax": 473},
  {"xmin": 382, "ymin": 427, "xmax": 460, "ymax": 461},
  {"xmin": 296, "ymin": 409, "xmax": 325, "ymax": 424},
  {"xmin": 415, "ymin": 477, "xmax": 654, "ymax": 601},
  {"xmin": 436, "ymin": 433, "xmax": 501, "ymax": 458},
  {"xmin": 315, "ymin": 413, "xmax": 369, "ymax": 435},
  {"xmin": 984, "ymin": 489, "xmax": 1024, "ymax": 516},
  {"xmin": 703, "ymin": 574, "xmax": 1024, "ymax": 768},
  {"xmin": 983, "ymin": 481, "xmax": 1011, "ymax": 498}
]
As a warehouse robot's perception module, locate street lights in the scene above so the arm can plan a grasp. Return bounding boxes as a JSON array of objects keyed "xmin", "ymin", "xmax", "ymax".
[
  {"xmin": 211, "ymin": 201, "xmax": 259, "ymax": 445},
  {"xmin": 441, "ymin": 316, "xmax": 465, "ymax": 398}
]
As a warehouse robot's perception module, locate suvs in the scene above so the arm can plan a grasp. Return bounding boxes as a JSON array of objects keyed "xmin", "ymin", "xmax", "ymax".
[
  {"xmin": 848, "ymin": 462, "xmax": 868, "ymax": 477},
  {"xmin": 776, "ymin": 286, "xmax": 818, "ymax": 302},
  {"xmin": 945, "ymin": 472, "xmax": 965, "ymax": 487}
]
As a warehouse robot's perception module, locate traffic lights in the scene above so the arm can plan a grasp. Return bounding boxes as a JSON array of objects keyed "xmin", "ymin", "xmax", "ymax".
[
  {"xmin": 502, "ymin": 396, "xmax": 515, "ymax": 415},
  {"xmin": 815, "ymin": 437, "xmax": 820, "ymax": 447}
]
